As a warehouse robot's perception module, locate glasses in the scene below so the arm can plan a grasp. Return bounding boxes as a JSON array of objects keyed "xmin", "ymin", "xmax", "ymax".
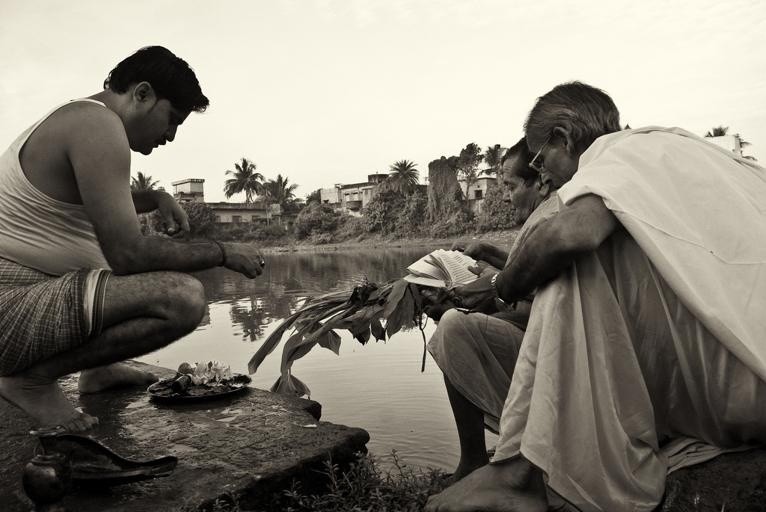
[{"xmin": 527, "ymin": 132, "xmax": 555, "ymax": 174}]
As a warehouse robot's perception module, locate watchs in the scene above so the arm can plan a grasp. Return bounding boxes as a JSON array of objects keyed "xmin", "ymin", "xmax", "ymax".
[{"xmin": 490, "ymin": 271, "xmax": 512, "ymax": 313}]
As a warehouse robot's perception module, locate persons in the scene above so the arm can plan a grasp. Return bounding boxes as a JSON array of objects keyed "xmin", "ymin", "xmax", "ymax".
[
  {"xmin": 1, "ymin": 44, "xmax": 266, "ymax": 435},
  {"xmin": 420, "ymin": 134, "xmax": 558, "ymax": 488},
  {"xmin": 421, "ymin": 80, "xmax": 766, "ymax": 512}
]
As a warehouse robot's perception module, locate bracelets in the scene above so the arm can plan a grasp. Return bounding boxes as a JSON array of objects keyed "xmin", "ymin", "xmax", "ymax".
[{"xmin": 211, "ymin": 240, "xmax": 226, "ymax": 267}]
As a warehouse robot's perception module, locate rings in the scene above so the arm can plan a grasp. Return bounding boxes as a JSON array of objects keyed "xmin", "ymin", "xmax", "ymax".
[{"xmin": 259, "ymin": 258, "xmax": 264, "ymax": 266}]
